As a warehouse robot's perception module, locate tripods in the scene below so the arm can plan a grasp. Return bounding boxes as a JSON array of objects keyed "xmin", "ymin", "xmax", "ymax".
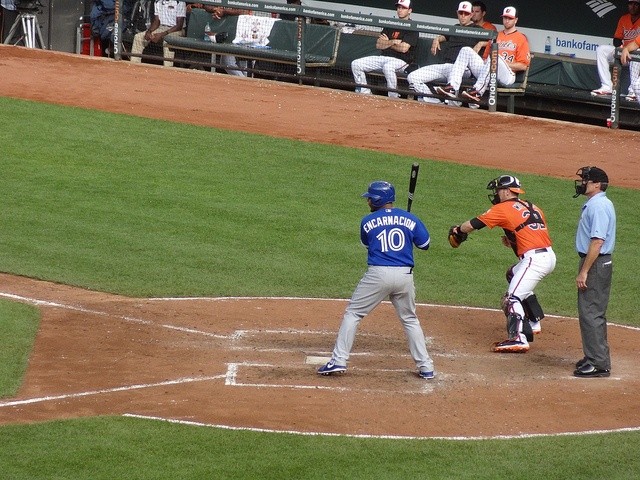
[{"xmin": 4, "ymin": 12, "xmax": 47, "ymax": 50}]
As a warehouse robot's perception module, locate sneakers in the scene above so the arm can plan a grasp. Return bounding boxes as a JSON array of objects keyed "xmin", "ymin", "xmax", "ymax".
[
  {"xmin": 317, "ymin": 361, "xmax": 346, "ymax": 375},
  {"xmin": 576, "ymin": 357, "xmax": 588, "ymax": 369},
  {"xmin": 437, "ymin": 83, "xmax": 456, "ymax": 100},
  {"xmin": 529, "ymin": 319, "xmax": 541, "ymax": 335},
  {"xmin": 625, "ymin": 96, "xmax": 637, "ymax": 102},
  {"xmin": 491, "ymin": 334, "xmax": 529, "ymax": 353},
  {"xmin": 574, "ymin": 364, "xmax": 610, "ymax": 377},
  {"xmin": 591, "ymin": 90, "xmax": 612, "ymax": 100},
  {"xmin": 419, "ymin": 371, "xmax": 434, "ymax": 379},
  {"xmin": 462, "ymin": 88, "xmax": 481, "ymax": 101}
]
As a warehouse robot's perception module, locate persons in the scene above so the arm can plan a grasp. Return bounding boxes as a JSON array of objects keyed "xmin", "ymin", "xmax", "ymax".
[
  {"xmin": 317, "ymin": 180, "xmax": 435, "ymax": 379},
  {"xmin": 130, "ymin": 0, "xmax": 187, "ymax": 66},
  {"xmin": 407, "ymin": 2, "xmax": 488, "ymax": 104},
  {"xmin": 590, "ymin": 0, "xmax": 640, "ymax": 103},
  {"xmin": 448, "ymin": 174, "xmax": 556, "ymax": 353},
  {"xmin": 437, "ymin": 6, "xmax": 531, "ymax": 103},
  {"xmin": 202, "ymin": 4, "xmax": 249, "ymax": 77},
  {"xmin": 471, "ymin": 1, "xmax": 497, "ymax": 62},
  {"xmin": 350, "ymin": 0, "xmax": 419, "ymax": 98},
  {"xmin": 574, "ymin": 166, "xmax": 616, "ymax": 378},
  {"xmin": 620, "ymin": 34, "xmax": 640, "ymax": 103}
]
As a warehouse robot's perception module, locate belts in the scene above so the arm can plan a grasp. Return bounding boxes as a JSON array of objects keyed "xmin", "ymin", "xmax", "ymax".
[
  {"xmin": 578, "ymin": 252, "xmax": 611, "ymax": 257},
  {"xmin": 521, "ymin": 248, "xmax": 547, "ymax": 259}
]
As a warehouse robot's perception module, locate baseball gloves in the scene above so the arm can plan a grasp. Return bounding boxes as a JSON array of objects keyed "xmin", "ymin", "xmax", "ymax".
[{"xmin": 449, "ymin": 225, "xmax": 468, "ymax": 248}]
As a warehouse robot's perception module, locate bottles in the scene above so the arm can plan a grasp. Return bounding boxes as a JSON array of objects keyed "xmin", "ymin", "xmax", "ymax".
[{"xmin": 543, "ymin": 34, "xmax": 553, "ymax": 56}]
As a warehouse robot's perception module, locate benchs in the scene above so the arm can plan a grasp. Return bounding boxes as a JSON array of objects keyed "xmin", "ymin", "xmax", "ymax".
[
  {"xmin": 162, "ymin": 8, "xmax": 341, "ymax": 80},
  {"xmin": 336, "ymin": 33, "xmax": 528, "ymax": 93},
  {"xmin": 527, "ymin": 57, "xmax": 640, "ymax": 112}
]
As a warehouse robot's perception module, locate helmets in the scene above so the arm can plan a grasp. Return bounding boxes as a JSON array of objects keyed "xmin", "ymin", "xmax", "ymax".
[
  {"xmin": 573, "ymin": 166, "xmax": 608, "ymax": 198},
  {"xmin": 361, "ymin": 181, "xmax": 395, "ymax": 207},
  {"xmin": 487, "ymin": 175, "xmax": 525, "ymax": 205}
]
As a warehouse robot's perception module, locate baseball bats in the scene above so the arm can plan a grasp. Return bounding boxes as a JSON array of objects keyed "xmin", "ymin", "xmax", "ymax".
[{"xmin": 407, "ymin": 162, "xmax": 419, "ymax": 212}]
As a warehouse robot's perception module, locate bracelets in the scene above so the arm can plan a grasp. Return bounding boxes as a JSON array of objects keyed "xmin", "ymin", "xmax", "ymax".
[{"xmin": 166, "ymin": 31, "xmax": 169, "ymax": 34}]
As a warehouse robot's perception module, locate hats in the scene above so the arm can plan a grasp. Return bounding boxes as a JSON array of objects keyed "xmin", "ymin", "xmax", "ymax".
[
  {"xmin": 501, "ymin": 6, "xmax": 519, "ymax": 18},
  {"xmin": 394, "ymin": 0, "xmax": 413, "ymax": 9},
  {"xmin": 457, "ymin": 1, "xmax": 473, "ymax": 15}
]
